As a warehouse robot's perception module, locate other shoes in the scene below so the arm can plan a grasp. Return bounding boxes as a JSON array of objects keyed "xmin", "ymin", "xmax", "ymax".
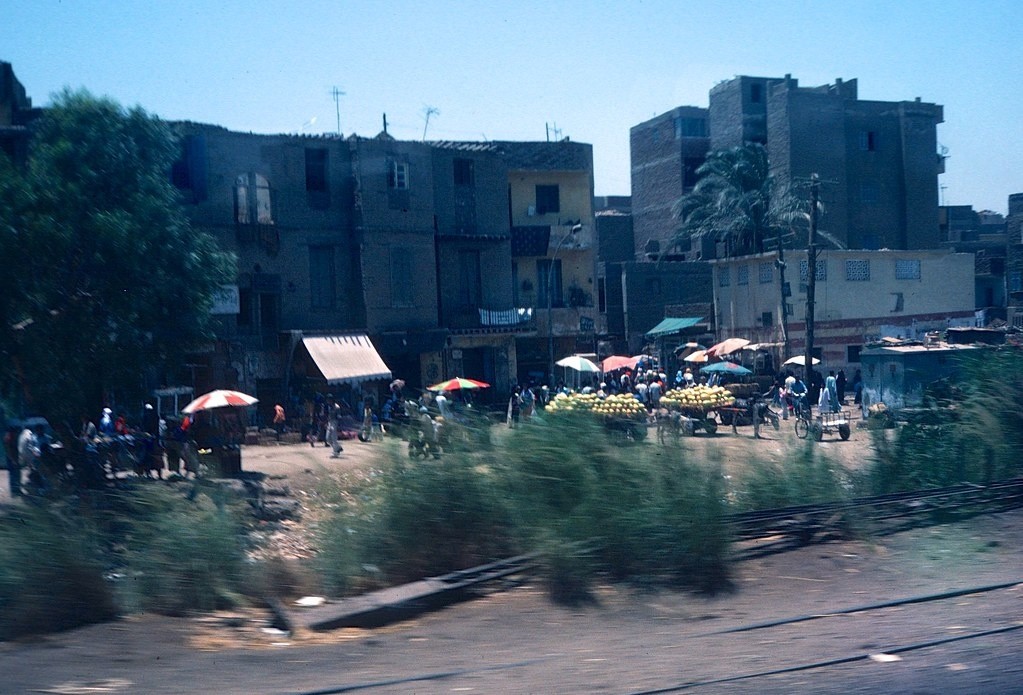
[
  {"xmin": 330, "ymin": 452, "xmax": 340, "ymax": 457},
  {"xmin": 858, "ymin": 406, "xmax": 862, "ymax": 409}
]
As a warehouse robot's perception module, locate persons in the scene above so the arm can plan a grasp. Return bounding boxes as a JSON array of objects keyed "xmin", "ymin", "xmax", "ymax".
[
  {"xmin": 273, "ymin": 378, "xmax": 488, "ymax": 460},
  {"xmin": 508, "ymin": 364, "xmax": 864, "ymax": 442},
  {"xmin": 3, "ymin": 423, "xmax": 56, "ymax": 498},
  {"xmin": 79, "ymin": 403, "xmax": 241, "ymax": 479}
]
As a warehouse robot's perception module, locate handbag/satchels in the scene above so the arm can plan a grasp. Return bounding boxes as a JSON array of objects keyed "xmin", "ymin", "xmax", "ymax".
[{"xmin": 645, "ymin": 392, "xmax": 650, "ymax": 402}]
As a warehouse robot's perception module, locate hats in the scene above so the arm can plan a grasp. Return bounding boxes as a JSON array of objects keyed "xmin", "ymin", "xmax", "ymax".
[
  {"xmin": 677, "ymin": 371, "xmax": 682, "ymax": 377},
  {"xmin": 686, "ymin": 368, "xmax": 690, "ymax": 372},
  {"xmin": 639, "ymin": 377, "xmax": 644, "ymax": 383},
  {"xmin": 638, "ymin": 367, "xmax": 643, "ymax": 371}
]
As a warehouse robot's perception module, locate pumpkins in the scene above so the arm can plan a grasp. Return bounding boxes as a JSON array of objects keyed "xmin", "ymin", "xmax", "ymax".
[
  {"xmin": 659, "ymin": 384, "xmax": 735, "ymax": 407},
  {"xmin": 545, "ymin": 393, "xmax": 647, "ymax": 415}
]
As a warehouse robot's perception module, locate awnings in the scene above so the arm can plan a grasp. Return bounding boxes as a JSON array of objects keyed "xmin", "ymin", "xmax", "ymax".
[
  {"xmin": 302, "ymin": 333, "xmax": 392, "ymax": 386},
  {"xmin": 638, "ymin": 318, "xmax": 707, "ymax": 344}
]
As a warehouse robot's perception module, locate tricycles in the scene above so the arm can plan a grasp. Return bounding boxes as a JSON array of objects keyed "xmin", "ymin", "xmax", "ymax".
[{"xmin": 793, "ymin": 396, "xmax": 852, "ymax": 442}]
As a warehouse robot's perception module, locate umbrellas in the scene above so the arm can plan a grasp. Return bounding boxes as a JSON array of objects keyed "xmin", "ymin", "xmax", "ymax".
[
  {"xmin": 673, "ymin": 336, "xmax": 821, "ymax": 376},
  {"xmin": 181, "ymin": 389, "xmax": 259, "ymax": 415},
  {"xmin": 555, "ymin": 355, "xmax": 658, "ymax": 373},
  {"xmin": 426, "ymin": 376, "xmax": 491, "ymax": 392}
]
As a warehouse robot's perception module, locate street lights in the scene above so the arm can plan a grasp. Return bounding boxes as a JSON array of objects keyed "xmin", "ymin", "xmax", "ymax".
[{"xmin": 546, "ymin": 223, "xmax": 583, "ymax": 392}]
